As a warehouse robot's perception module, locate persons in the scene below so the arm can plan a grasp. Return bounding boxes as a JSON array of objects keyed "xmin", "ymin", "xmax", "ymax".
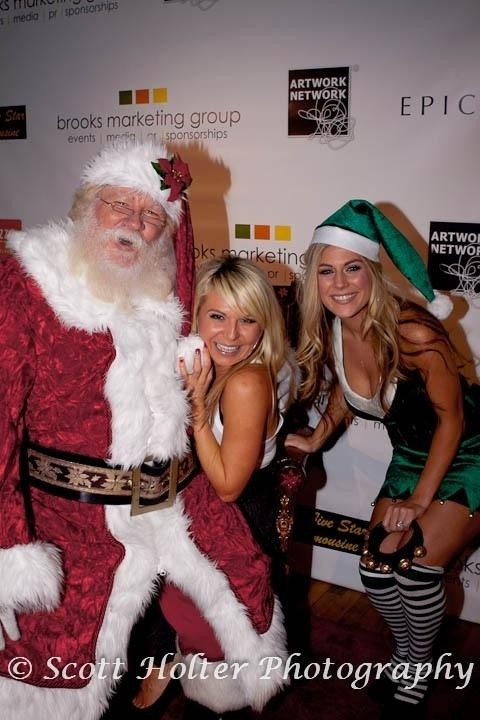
[
  {"xmin": 132, "ymin": 254, "xmax": 289, "ymax": 709},
  {"xmin": 1, "ymin": 136, "xmax": 293, "ymax": 719},
  {"xmin": 282, "ymin": 200, "xmax": 479, "ymax": 720}
]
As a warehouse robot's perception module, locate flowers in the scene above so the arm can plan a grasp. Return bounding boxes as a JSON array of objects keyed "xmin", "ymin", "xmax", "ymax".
[{"xmin": 151, "ymin": 153, "xmax": 194, "ymax": 202}]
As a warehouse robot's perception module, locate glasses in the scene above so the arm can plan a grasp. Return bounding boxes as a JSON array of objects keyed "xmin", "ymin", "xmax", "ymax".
[{"xmin": 99, "ymin": 197, "xmax": 164, "ymax": 228}]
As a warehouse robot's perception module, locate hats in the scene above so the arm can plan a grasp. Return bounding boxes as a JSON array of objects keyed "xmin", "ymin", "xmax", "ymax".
[
  {"xmin": 88, "ymin": 143, "xmax": 213, "ymax": 374},
  {"xmin": 310, "ymin": 200, "xmax": 454, "ymax": 320}
]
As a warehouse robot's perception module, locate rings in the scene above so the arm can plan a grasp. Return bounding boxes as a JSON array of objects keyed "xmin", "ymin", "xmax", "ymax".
[{"xmin": 396, "ymin": 520, "xmax": 403, "ymax": 527}]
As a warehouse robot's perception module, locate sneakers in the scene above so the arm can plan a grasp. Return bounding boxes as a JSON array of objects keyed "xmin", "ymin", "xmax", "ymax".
[{"xmin": 368, "ymin": 661, "xmax": 440, "ymax": 720}]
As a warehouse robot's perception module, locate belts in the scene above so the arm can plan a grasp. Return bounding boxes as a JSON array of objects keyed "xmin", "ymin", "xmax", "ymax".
[{"xmin": 23, "ymin": 443, "xmax": 200, "ymax": 515}]
